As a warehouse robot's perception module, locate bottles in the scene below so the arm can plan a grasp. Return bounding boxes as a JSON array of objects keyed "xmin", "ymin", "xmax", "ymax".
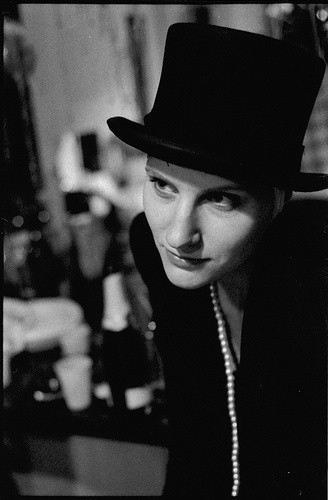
[{"xmin": 98, "ymin": 265, "xmax": 159, "ymax": 433}]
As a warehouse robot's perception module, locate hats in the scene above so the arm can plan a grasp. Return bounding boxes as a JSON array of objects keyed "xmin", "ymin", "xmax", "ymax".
[{"xmin": 107, "ymin": 21, "xmax": 328, "ymax": 193}]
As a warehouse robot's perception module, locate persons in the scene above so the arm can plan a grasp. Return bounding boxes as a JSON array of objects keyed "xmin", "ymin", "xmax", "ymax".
[
  {"xmin": 107, "ymin": 20, "xmax": 328, "ymax": 500},
  {"xmin": 60, "ymin": 134, "xmax": 165, "ymax": 415}
]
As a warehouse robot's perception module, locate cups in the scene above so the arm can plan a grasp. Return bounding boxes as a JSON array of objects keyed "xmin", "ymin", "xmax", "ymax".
[{"xmin": 55, "ymin": 356, "xmax": 95, "ymax": 414}]
